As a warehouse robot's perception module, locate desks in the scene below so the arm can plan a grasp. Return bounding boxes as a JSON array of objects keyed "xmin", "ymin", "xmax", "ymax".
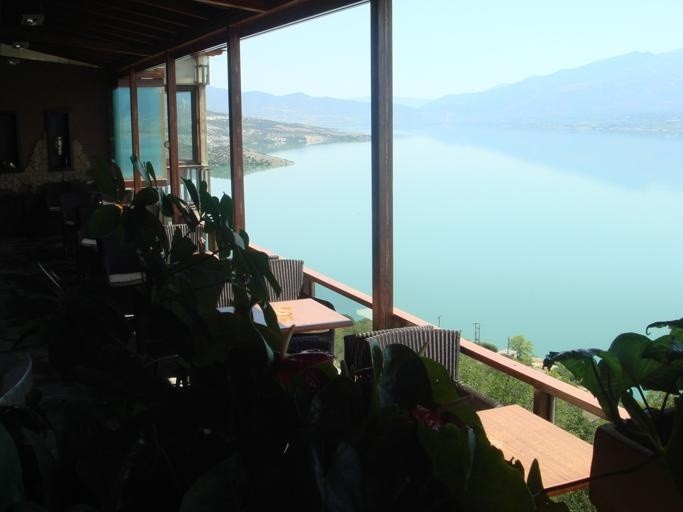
[
  {"xmin": 254, "ymin": 298, "xmax": 352, "ymax": 336},
  {"xmin": 0, "ymin": 190, "xmax": 33, "ymax": 239},
  {"xmin": 476, "ymin": 403, "xmax": 595, "ymax": 499}
]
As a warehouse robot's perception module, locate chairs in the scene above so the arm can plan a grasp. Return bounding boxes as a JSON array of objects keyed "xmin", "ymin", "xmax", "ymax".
[
  {"xmin": 43, "ymin": 184, "xmax": 72, "ymax": 228},
  {"xmin": 343, "ymin": 325, "xmax": 433, "ymax": 373},
  {"xmin": 163, "ymin": 223, "xmax": 203, "ymax": 254},
  {"xmin": 217, "ymin": 258, "xmax": 303, "ymax": 308},
  {"xmin": 60, "ymin": 193, "xmax": 90, "ymax": 240},
  {"xmin": 366, "ymin": 330, "xmax": 460, "ymax": 387}
]
